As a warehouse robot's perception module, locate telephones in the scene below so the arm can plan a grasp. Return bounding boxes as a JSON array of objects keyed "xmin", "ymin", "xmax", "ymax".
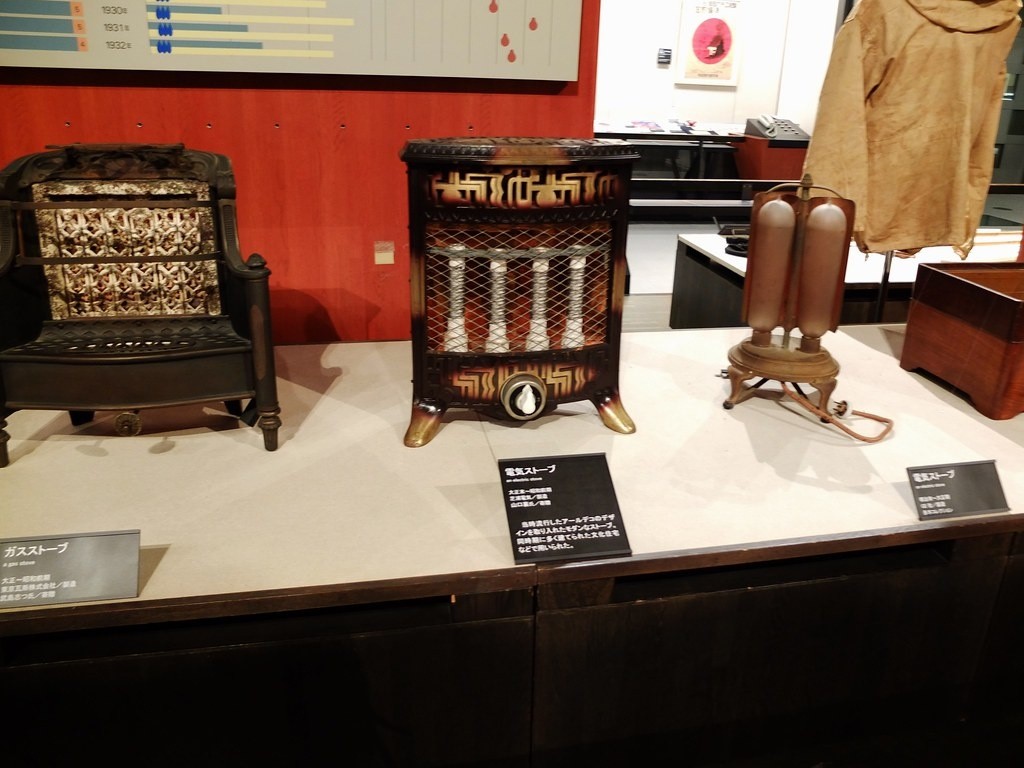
[{"xmin": 758, "ymin": 112, "xmax": 775, "ymax": 130}]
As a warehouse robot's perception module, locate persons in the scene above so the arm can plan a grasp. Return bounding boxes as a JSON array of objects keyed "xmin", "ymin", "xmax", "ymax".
[{"xmin": 795, "ymin": 0, "xmax": 1024, "ymax": 261}]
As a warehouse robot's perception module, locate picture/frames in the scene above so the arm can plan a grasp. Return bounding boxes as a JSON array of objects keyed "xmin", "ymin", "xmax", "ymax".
[{"xmin": 674, "ymin": 1, "xmax": 746, "ymax": 87}]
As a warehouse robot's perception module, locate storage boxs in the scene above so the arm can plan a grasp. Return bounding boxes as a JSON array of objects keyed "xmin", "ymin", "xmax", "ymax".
[{"xmin": 899, "ymin": 262, "xmax": 1024, "ymax": 420}]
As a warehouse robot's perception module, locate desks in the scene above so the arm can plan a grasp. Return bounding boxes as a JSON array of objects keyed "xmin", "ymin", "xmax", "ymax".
[
  {"xmin": 670, "ymin": 225, "xmax": 1024, "ymax": 329},
  {"xmin": 594, "ymin": 122, "xmax": 745, "ymax": 179},
  {"xmin": 1, "ymin": 319, "xmax": 1024, "ymax": 768}
]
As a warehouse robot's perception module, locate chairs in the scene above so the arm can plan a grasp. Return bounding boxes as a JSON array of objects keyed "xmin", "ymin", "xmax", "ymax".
[{"xmin": 0, "ymin": 143, "xmax": 281, "ymax": 469}]
